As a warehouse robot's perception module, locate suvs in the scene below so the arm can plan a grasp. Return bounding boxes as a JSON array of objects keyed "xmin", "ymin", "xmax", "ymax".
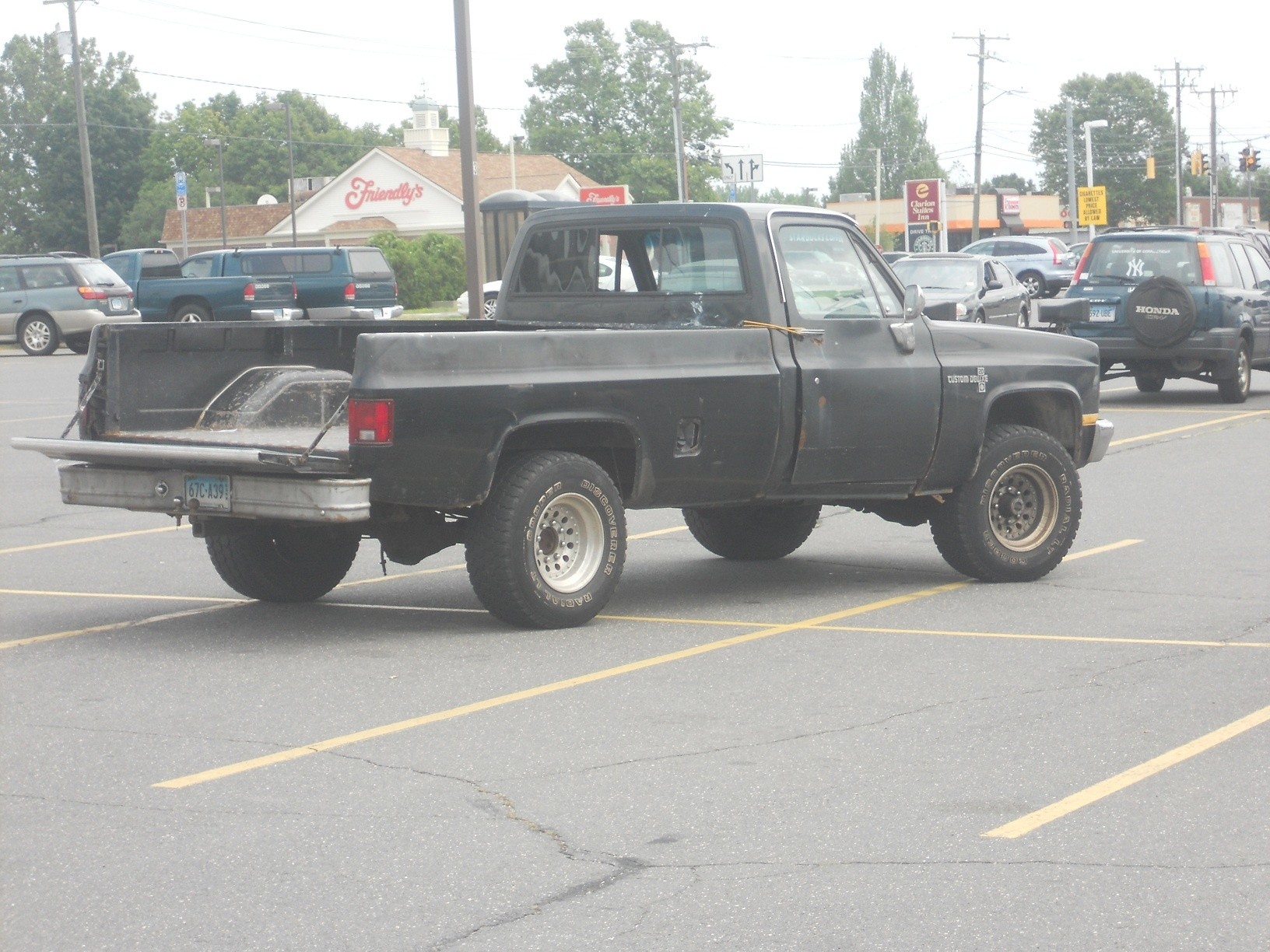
[
  {"xmin": 953, "ymin": 233, "xmax": 1081, "ymax": 300},
  {"xmin": 177, "ymin": 245, "xmax": 406, "ymax": 323},
  {"xmin": 1036, "ymin": 229, "xmax": 1270, "ymax": 404},
  {"xmin": 0, "ymin": 249, "xmax": 143, "ymax": 358}
]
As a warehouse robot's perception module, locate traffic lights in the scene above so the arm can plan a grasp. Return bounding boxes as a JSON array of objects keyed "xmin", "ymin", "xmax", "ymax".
[
  {"xmin": 1240, "ymin": 158, "xmax": 1248, "ymax": 173},
  {"xmin": 1251, "ymin": 149, "xmax": 1262, "ymax": 169},
  {"xmin": 1197, "ymin": 149, "xmax": 1209, "ymax": 175},
  {"xmin": 1247, "ymin": 157, "xmax": 1255, "ymax": 171}
]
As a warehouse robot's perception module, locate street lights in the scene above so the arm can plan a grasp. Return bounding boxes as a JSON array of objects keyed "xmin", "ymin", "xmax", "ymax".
[
  {"xmin": 204, "ymin": 138, "xmax": 227, "ymax": 249},
  {"xmin": 509, "ymin": 132, "xmax": 524, "ymax": 190},
  {"xmin": 970, "ymin": 88, "xmax": 1029, "ymax": 243},
  {"xmin": 867, "ymin": 148, "xmax": 882, "ymax": 246},
  {"xmin": 1084, "ymin": 118, "xmax": 1109, "ymax": 241},
  {"xmin": 264, "ymin": 101, "xmax": 297, "ymax": 245}
]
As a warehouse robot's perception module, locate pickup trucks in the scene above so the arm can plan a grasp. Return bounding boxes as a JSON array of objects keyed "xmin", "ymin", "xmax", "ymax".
[
  {"xmin": 95, "ymin": 247, "xmax": 299, "ymax": 324},
  {"xmin": 8, "ymin": 199, "xmax": 1118, "ymax": 633}
]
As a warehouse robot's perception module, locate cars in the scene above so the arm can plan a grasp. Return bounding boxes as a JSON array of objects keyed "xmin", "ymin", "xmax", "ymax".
[
  {"xmin": 888, "ymin": 250, "xmax": 1032, "ymax": 330},
  {"xmin": 655, "ymin": 226, "xmax": 1270, "ymax": 330},
  {"xmin": 455, "ymin": 255, "xmax": 646, "ymax": 320}
]
